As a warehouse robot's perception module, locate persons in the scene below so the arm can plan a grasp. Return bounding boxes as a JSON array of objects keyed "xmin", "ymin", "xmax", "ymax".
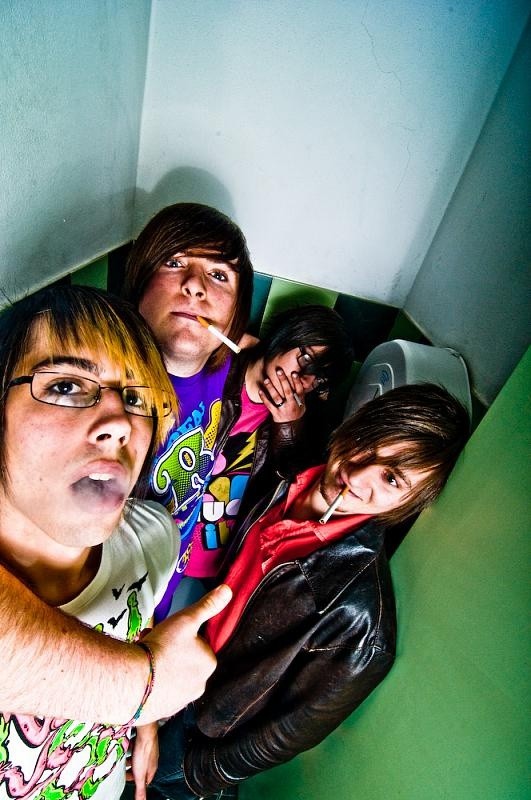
[
  {"xmin": 121, "ymin": 383, "xmax": 472, "ymax": 800},
  {"xmin": 0, "ymin": 283, "xmax": 182, "ymax": 800},
  {"xmin": 147, "ymin": 305, "xmax": 354, "ymax": 628},
  {"xmin": 0, "ymin": 203, "xmax": 255, "ymax": 727}
]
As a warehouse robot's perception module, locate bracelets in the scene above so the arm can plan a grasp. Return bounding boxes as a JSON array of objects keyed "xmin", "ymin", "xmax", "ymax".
[{"xmin": 124, "ymin": 642, "xmax": 156, "ymax": 728}]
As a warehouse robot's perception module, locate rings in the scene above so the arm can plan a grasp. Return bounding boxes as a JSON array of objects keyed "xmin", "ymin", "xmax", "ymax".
[{"xmin": 277, "ymin": 400, "xmax": 285, "ymax": 407}]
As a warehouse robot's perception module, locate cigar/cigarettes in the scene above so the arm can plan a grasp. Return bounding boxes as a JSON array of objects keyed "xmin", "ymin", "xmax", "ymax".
[
  {"xmin": 196, "ymin": 315, "xmax": 242, "ymax": 354},
  {"xmin": 319, "ymin": 485, "xmax": 351, "ymax": 524},
  {"xmin": 293, "ymin": 392, "xmax": 303, "ymax": 407}
]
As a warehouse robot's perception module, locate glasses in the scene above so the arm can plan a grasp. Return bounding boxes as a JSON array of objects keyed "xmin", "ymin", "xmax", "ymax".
[
  {"xmin": 8, "ymin": 372, "xmax": 172, "ymax": 419},
  {"xmin": 296, "ymin": 346, "xmax": 331, "ymax": 395}
]
